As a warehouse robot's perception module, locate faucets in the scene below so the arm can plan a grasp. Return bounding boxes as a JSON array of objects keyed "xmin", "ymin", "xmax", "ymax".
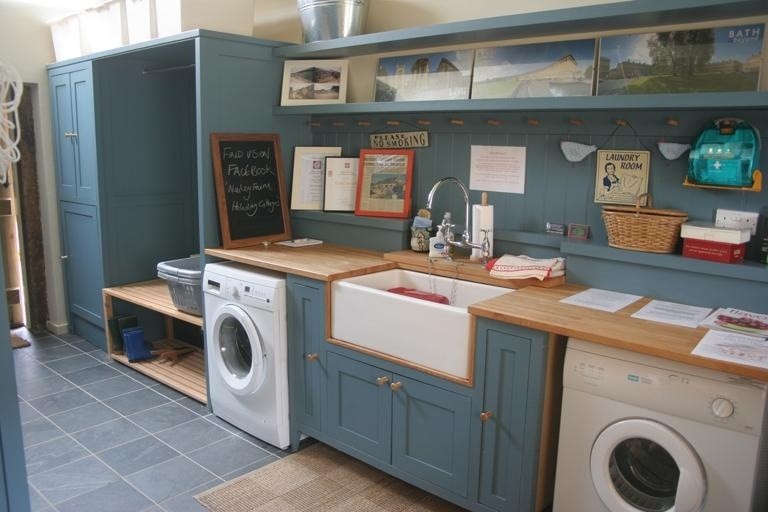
[{"xmin": 426, "ymin": 176, "xmax": 482, "ymax": 257}]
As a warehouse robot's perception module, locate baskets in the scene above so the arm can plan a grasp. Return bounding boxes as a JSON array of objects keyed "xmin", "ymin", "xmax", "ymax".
[
  {"xmin": 156, "ymin": 256, "xmax": 201, "ymax": 317},
  {"xmin": 594, "ymin": 191, "xmax": 690, "ymax": 256}
]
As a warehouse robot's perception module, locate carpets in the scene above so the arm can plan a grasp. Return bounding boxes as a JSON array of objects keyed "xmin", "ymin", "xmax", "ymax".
[
  {"xmin": 10, "ymin": 333, "xmax": 31, "ymax": 350},
  {"xmin": 191, "ymin": 443, "xmax": 469, "ymax": 512}
]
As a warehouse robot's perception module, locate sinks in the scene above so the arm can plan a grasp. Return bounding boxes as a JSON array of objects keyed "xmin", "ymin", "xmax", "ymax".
[{"xmin": 330, "ymin": 261, "xmax": 516, "ymax": 380}]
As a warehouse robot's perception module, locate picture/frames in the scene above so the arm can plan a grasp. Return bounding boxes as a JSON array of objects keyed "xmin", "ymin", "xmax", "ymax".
[
  {"xmin": 371, "ymin": 49, "xmax": 474, "ymax": 102},
  {"xmin": 596, "ymin": 24, "xmax": 764, "ymax": 94},
  {"xmin": 281, "ymin": 60, "xmax": 348, "ymax": 105},
  {"xmin": 594, "ymin": 149, "xmax": 651, "ymax": 207},
  {"xmin": 472, "ymin": 37, "xmax": 595, "ymax": 100},
  {"xmin": 288, "ymin": 144, "xmax": 416, "ymax": 220}
]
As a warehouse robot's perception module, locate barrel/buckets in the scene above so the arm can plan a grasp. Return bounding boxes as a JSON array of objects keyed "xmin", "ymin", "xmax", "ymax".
[{"xmin": 296, "ymin": 0, "xmax": 366, "ymax": 44}]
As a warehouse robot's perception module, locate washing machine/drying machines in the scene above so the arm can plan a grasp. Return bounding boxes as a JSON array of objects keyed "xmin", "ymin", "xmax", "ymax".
[
  {"xmin": 551, "ymin": 336, "xmax": 768, "ymax": 511},
  {"xmin": 201, "ymin": 262, "xmax": 291, "ymax": 451}
]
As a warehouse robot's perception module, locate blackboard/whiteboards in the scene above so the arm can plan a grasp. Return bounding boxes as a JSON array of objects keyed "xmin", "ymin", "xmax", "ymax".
[{"xmin": 210, "ymin": 133, "xmax": 292, "ymax": 250}]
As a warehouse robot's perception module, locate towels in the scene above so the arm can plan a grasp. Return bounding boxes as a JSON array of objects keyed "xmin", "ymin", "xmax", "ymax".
[{"xmin": 488, "ymin": 253, "xmax": 566, "ymax": 281}]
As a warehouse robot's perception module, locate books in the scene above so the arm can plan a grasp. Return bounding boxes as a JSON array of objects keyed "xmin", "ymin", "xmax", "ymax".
[{"xmin": 700, "ymin": 306, "xmax": 768, "ymax": 340}]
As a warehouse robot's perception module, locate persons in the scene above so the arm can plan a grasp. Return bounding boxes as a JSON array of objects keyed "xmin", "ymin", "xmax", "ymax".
[{"xmin": 602, "ymin": 162, "xmax": 619, "ymax": 191}]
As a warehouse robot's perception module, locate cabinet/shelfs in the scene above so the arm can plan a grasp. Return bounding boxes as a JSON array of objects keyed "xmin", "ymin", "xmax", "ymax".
[
  {"xmin": 470, "ymin": 305, "xmax": 549, "ymax": 512},
  {"xmin": 46, "ymin": 58, "xmax": 109, "ymax": 352},
  {"xmin": 321, "ymin": 339, "xmax": 470, "ymax": 512},
  {"xmin": 90, "ymin": 32, "xmax": 305, "ymax": 329},
  {"xmin": 273, "ymin": 0, "xmax": 768, "ymax": 116},
  {"xmin": 285, "ymin": 269, "xmax": 327, "ymax": 453},
  {"xmin": 101, "ymin": 278, "xmax": 208, "ymax": 406}
]
